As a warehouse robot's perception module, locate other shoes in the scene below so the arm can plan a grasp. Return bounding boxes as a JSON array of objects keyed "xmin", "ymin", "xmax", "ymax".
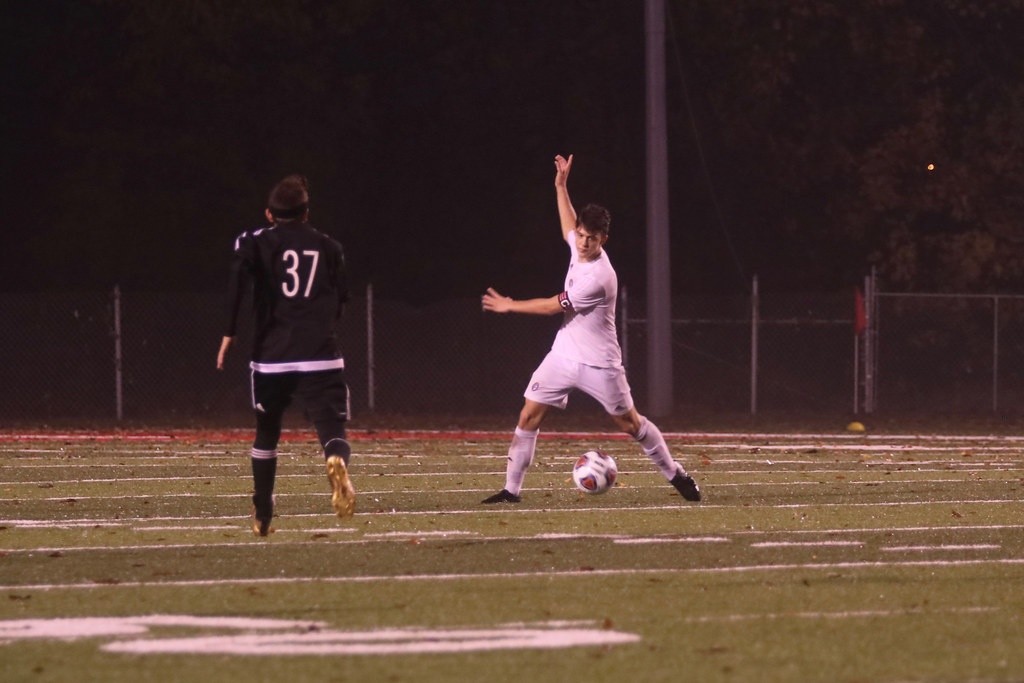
[
  {"xmin": 326, "ymin": 457, "xmax": 357, "ymax": 519},
  {"xmin": 251, "ymin": 502, "xmax": 272, "ymax": 536}
]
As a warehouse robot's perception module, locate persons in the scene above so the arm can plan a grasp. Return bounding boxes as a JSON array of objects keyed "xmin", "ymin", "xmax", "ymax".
[
  {"xmin": 483, "ymin": 154, "xmax": 701, "ymax": 503},
  {"xmin": 215, "ymin": 173, "xmax": 356, "ymax": 535}
]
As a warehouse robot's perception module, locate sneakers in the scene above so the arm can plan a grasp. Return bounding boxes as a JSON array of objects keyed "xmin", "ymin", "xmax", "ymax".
[
  {"xmin": 481, "ymin": 490, "xmax": 520, "ymax": 505},
  {"xmin": 670, "ymin": 472, "xmax": 701, "ymax": 501}
]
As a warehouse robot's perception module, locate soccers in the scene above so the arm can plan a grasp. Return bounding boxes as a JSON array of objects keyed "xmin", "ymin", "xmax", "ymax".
[{"xmin": 571, "ymin": 448, "xmax": 618, "ymax": 496}]
